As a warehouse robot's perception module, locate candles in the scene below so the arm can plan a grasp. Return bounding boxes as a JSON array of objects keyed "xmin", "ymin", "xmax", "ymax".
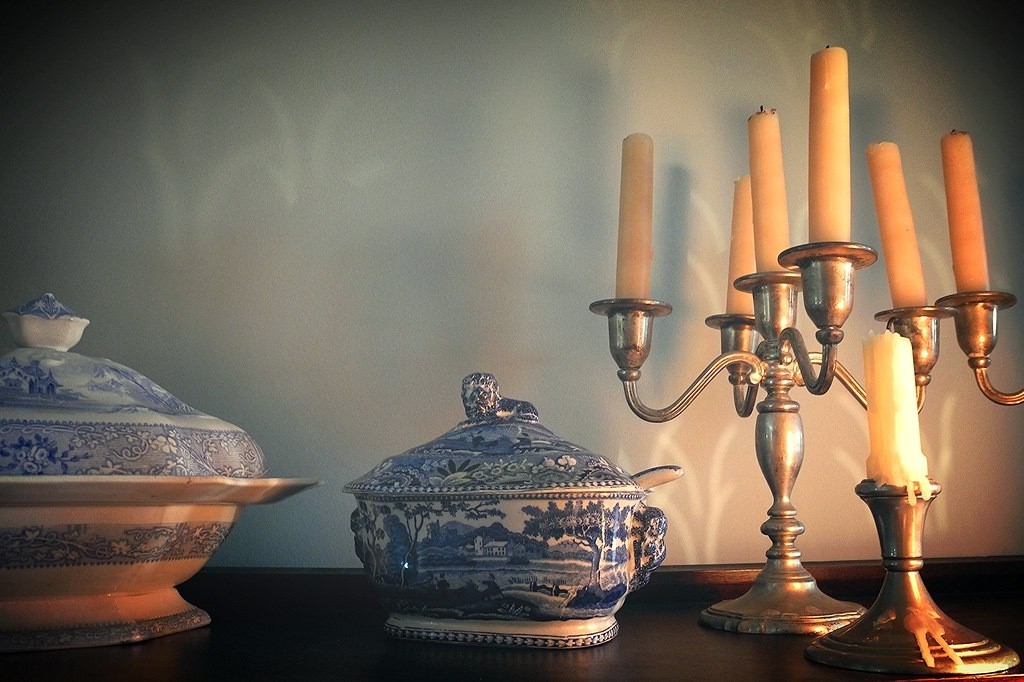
[
  {"xmin": 865, "ymin": 141, "xmax": 928, "ymax": 309},
  {"xmin": 747, "ymin": 104, "xmax": 791, "ymax": 274},
  {"xmin": 725, "ymin": 174, "xmax": 757, "ymax": 316},
  {"xmin": 614, "ymin": 132, "xmax": 654, "ymax": 300},
  {"xmin": 861, "ymin": 328, "xmax": 931, "ymax": 488},
  {"xmin": 940, "ymin": 128, "xmax": 1000, "ymax": 294},
  {"xmin": 807, "ymin": 44, "xmax": 852, "ymax": 245}
]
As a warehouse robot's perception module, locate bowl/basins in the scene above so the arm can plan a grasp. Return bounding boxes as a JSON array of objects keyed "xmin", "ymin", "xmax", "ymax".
[
  {"xmin": 340, "ymin": 373, "xmax": 669, "ymax": 649},
  {"xmin": 0, "ymin": 293, "xmax": 321, "ymax": 652}
]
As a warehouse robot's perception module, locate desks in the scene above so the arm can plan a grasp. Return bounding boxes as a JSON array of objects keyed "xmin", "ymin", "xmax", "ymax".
[{"xmin": 0, "ymin": 601, "xmax": 1024, "ymax": 682}]
